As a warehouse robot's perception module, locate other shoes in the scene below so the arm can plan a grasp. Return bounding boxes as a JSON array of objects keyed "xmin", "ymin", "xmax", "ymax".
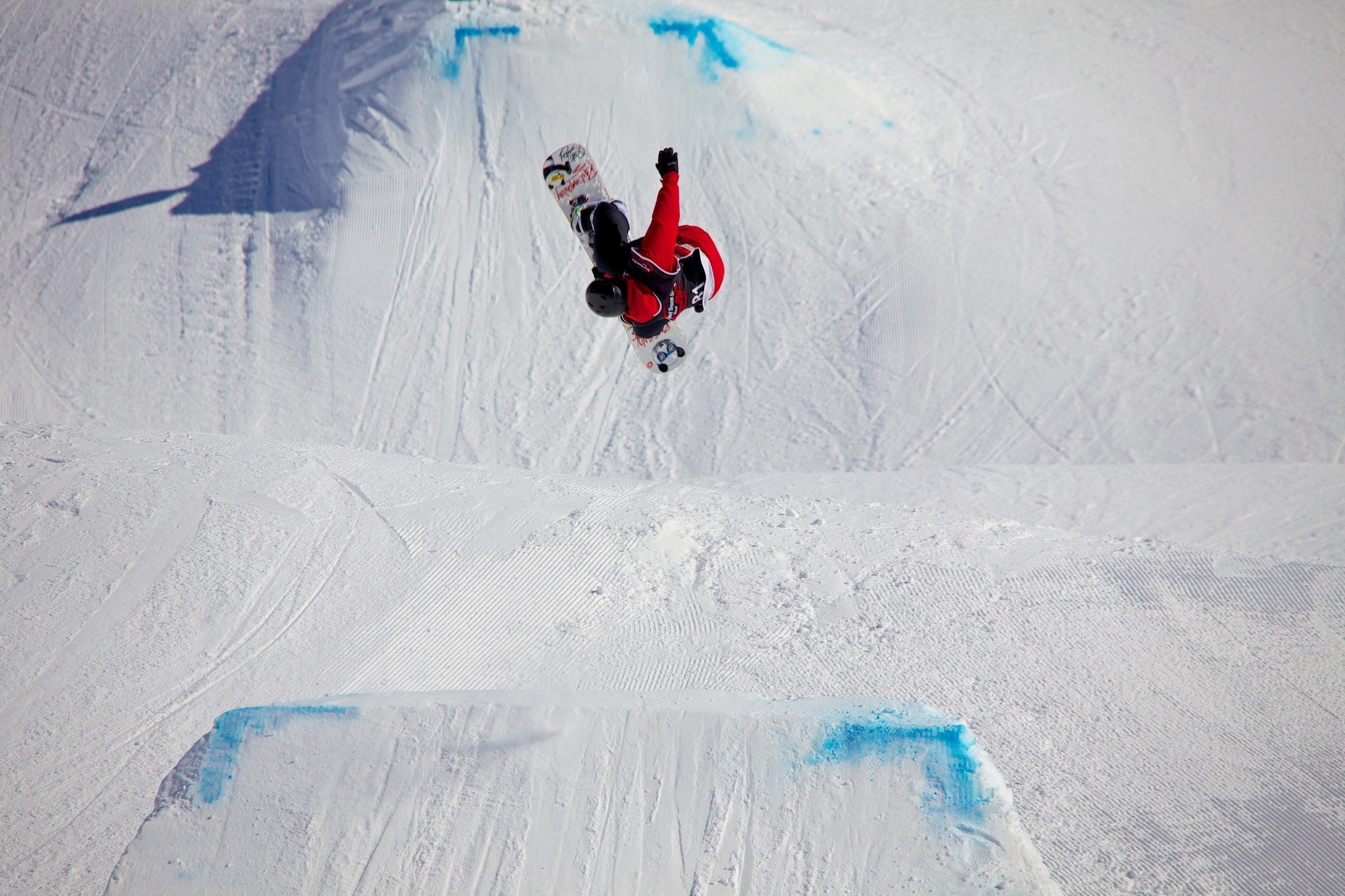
[{"xmin": 569, "ymin": 200, "xmax": 628, "ymax": 233}]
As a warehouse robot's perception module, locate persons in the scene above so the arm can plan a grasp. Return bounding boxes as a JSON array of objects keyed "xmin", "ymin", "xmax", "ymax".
[{"xmin": 569, "ymin": 147, "xmax": 725, "ymax": 341}]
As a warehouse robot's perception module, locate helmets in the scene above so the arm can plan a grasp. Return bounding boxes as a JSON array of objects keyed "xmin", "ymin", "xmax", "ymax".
[{"xmin": 585, "ymin": 279, "xmax": 626, "ymax": 316}]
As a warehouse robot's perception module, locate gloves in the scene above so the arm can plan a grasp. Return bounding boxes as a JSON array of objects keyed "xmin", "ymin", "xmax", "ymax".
[{"xmin": 656, "ymin": 147, "xmax": 678, "ymax": 178}]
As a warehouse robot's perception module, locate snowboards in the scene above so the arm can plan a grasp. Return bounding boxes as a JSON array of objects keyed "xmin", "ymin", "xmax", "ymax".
[{"xmin": 541, "ymin": 142, "xmax": 692, "ymax": 375}]
